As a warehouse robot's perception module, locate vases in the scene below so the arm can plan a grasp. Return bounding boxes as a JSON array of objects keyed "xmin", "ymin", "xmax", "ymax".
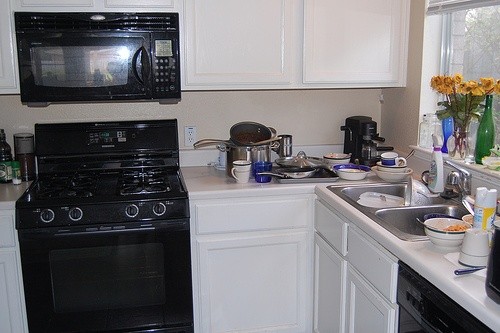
[{"xmin": 446, "ymin": 112, "xmax": 474, "ymax": 160}]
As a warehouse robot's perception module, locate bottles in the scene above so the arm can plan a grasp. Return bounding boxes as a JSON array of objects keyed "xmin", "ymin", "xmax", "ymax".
[
  {"xmin": 475, "ymin": 94, "xmax": 495, "ymax": 163},
  {"xmin": 474, "ymin": 185, "xmax": 498, "ymax": 249},
  {"xmin": 12, "ymin": 161, "xmax": 22, "ymax": 184},
  {"xmin": 0, "ymin": 128, "xmax": 14, "ymax": 184}
]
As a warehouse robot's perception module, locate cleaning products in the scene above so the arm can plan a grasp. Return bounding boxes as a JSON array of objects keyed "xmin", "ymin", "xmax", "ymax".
[{"xmin": 427, "ymin": 132, "xmax": 445, "ymax": 193}]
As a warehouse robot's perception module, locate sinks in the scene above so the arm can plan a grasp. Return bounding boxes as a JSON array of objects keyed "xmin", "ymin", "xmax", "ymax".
[
  {"xmin": 374, "ymin": 206, "xmax": 470, "ymax": 242},
  {"xmin": 326, "ymin": 182, "xmax": 445, "ymax": 208}
]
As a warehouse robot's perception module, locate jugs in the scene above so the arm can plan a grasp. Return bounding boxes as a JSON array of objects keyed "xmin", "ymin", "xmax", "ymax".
[{"xmin": 362, "ymin": 142, "xmax": 377, "ymax": 159}]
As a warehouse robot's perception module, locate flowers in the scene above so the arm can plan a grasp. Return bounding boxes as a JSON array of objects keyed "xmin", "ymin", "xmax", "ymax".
[{"xmin": 430, "ymin": 73, "xmax": 500, "ymax": 123}]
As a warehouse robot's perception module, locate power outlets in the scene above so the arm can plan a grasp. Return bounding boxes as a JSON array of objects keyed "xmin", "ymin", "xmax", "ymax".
[{"xmin": 184, "ymin": 127, "xmax": 197, "ymax": 147}]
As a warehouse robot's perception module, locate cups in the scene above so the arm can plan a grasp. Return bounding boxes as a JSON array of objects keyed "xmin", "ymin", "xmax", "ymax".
[
  {"xmin": 380, "ymin": 152, "xmax": 407, "ymax": 167},
  {"xmin": 277, "ymin": 134, "xmax": 293, "ymax": 156},
  {"xmin": 252, "ymin": 161, "xmax": 272, "ymax": 183},
  {"xmin": 231, "ymin": 160, "xmax": 252, "ymax": 183},
  {"xmin": 458, "ymin": 229, "xmax": 491, "ymax": 267},
  {"xmin": 13, "ymin": 132, "xmax": 36, "ymax": 182}
]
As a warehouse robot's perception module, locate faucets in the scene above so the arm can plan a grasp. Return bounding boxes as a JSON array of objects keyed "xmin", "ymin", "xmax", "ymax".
[{"xmin": 443, "ymin": 159, "xmax": 472, "ymax": 194}]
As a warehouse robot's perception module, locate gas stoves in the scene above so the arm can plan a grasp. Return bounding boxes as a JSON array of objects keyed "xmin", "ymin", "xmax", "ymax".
[{"xmin": 15, "ymin": 119, "xmax": 190, "ymax": 230}]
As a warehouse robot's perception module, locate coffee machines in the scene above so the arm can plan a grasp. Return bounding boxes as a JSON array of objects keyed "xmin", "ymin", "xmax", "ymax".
[{"xmin": 340, "ymin": 115, "xmax": 378, "ymax": 167}]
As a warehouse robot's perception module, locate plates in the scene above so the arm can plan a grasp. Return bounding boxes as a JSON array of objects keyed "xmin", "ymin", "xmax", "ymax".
[{"xmin": 275, "ymin": 156, "xmax": 325, "ymax": 168}]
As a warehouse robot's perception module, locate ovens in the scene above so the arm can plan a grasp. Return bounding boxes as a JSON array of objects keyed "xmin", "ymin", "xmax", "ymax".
[{"xmin": 18, "ymin": 217, "xmax": 194, "ymax": 333}]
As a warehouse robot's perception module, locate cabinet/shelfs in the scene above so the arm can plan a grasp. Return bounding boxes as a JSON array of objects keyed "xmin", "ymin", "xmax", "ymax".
[
  {"xmin": 0, "ymin": 0, "xmax": 410, "ymax": 94},
  {"xmin": 189, "ymin": 198, "xmax": 399, "ymax": 333},
  {"xmin": 0, "ymin": 210, "xmax": 29, "ymax": 333}
]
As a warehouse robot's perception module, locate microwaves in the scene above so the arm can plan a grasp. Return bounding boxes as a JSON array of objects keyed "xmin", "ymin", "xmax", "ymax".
[{"xmin": 14, "ymin": 11, "xmax": 181, "ymax": 108}]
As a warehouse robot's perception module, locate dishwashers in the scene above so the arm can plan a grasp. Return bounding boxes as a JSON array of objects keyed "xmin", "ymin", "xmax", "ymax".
[{"xmin": 397, "ymin": 261, "xmax": 495, "ymax": 333}]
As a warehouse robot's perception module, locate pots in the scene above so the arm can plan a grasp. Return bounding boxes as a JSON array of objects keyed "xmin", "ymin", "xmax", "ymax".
[
  {"xmin": 216, "ymin": 142, "xmax": 280, "ymax": 178},
  {"xmin": 193, "ymin": 120, "xmax": 276, "ymax": 150},
  {"xmin": 256, "ymin": 166, "xmax": 317, "ymax": 179}
]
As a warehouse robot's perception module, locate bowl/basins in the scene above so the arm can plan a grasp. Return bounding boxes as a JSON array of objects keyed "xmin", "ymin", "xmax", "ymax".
[
  {"xmin": 370, "ymin": 166, "xmax": 413, "ymax": 182},
  {"xmin": 332, "ymin": 163, "xmax": 372, "ymax": 181},
  {"xmin": 423, "ymin": 218, "xmax": 473, "ymax": 247},
  {"xmin": 322, "ymin": 154, "xmax": 351, "ymax": 167},
  {"xmin": 376, "ymin": 161, "xmax": 408, "ymax": 172}
]
